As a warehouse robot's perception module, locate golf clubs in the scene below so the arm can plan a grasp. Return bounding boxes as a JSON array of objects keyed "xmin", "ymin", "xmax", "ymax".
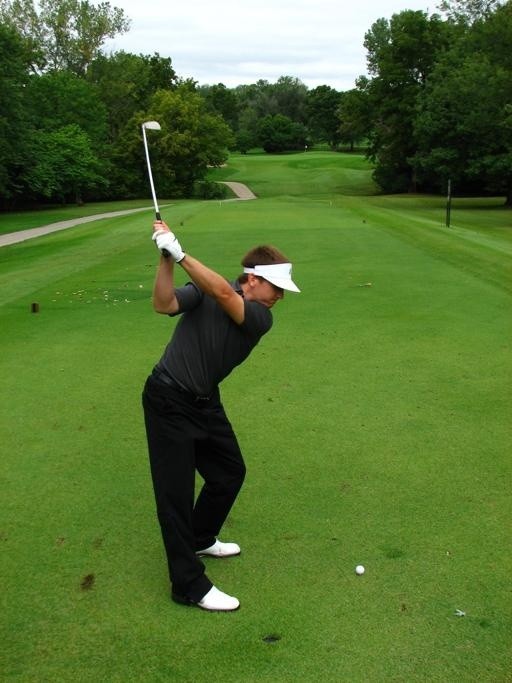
[{"xmin": 142, "ymin": 120, "xmax": 170, "ymax": 258}]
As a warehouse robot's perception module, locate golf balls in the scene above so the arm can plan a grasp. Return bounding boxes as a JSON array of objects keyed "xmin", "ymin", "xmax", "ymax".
[
  {"xmin": 354, "ymin": 565, "xmax": 365, "ymax": 574},
  {"xmin": 139, "ymin": 284, "xmax": 142, "ymax": 288}
]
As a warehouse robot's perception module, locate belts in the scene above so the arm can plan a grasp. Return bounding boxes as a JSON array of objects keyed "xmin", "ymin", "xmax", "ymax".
[{"xmin": 152, "ymin": 367, "xmax": 215, "ymax": 404}]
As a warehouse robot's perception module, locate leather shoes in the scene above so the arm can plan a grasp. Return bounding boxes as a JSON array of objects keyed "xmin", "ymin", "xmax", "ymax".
[
  {"xmin": 195, "ymin": 538, "xmax": 241, "ymax": 558},
  {"xmin": 171, "ymin": 583, "xmax": 240, "ymax": 611}
]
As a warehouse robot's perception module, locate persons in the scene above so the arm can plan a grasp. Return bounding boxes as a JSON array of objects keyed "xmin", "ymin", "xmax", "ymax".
[{"xmin": 140, "ymin": 221, "xmax": 302, "ymax": 612}]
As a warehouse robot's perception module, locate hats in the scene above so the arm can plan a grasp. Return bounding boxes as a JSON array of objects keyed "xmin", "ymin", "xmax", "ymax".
[{"xmin": 243, "ymin": 262, "xmax": 301, "ymax": 293}]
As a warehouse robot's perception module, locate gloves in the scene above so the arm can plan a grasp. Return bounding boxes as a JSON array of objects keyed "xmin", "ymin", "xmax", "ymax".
[{"xmin": 151, "ymin": 229, "xmax": 186, "ymax": 263}]
